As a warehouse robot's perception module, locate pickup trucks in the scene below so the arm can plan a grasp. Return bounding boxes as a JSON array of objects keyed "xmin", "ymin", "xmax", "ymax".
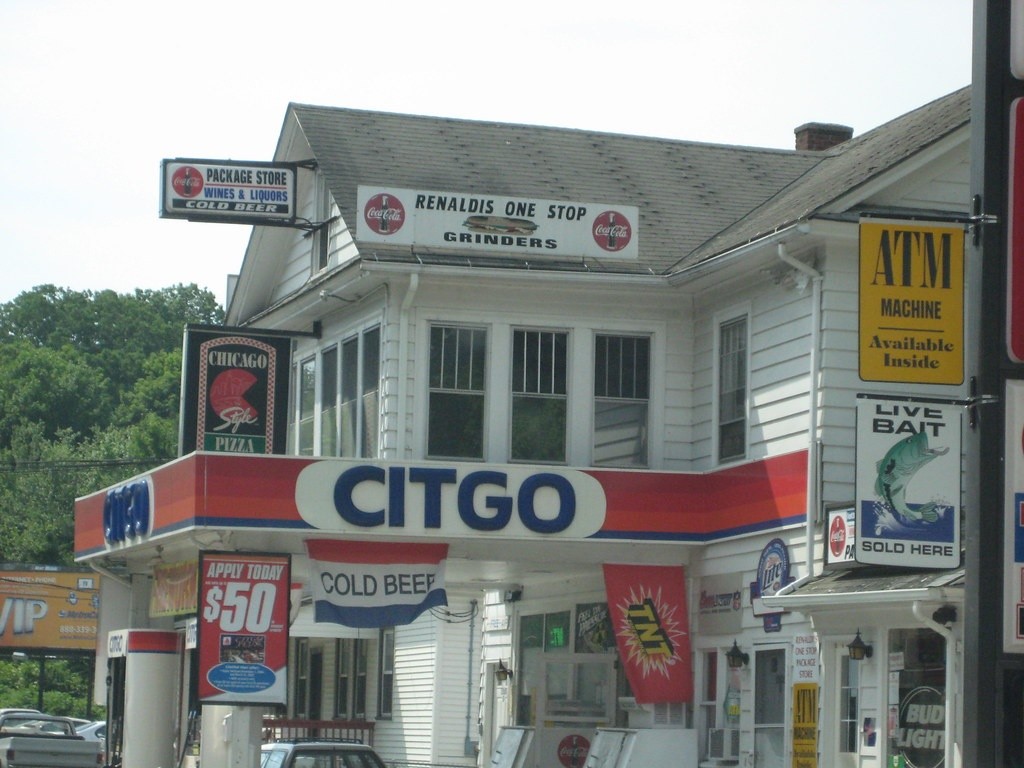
[{"xmin": 0, "ymin": 712, "xmax": 102, "ymax": 768}]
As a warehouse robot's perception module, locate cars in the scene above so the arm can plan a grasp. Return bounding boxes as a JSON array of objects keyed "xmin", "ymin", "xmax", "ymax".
[
  {"xmin": 14, "ymin": 715, "xmax": 106, "ymax": 750},
  {"xmin": 259, "ymin": 735, "xmax": 388, "ymax": 768},
  {"xmin": 0, "ymin": 709, "xmax": 40, "ymax": 718}
]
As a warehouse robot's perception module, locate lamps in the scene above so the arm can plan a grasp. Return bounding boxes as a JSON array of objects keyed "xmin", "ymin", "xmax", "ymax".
[
  {"xmin": 724, "ymin": 638, "xmax": 750, "ymax": 668},
  {"xmin": 846, "ymin": 627, "xmax": 873, "ymax": 661},
  {"xmin": 494, "ymin": 657, "xmax": 512, "ymax": 681}
]
